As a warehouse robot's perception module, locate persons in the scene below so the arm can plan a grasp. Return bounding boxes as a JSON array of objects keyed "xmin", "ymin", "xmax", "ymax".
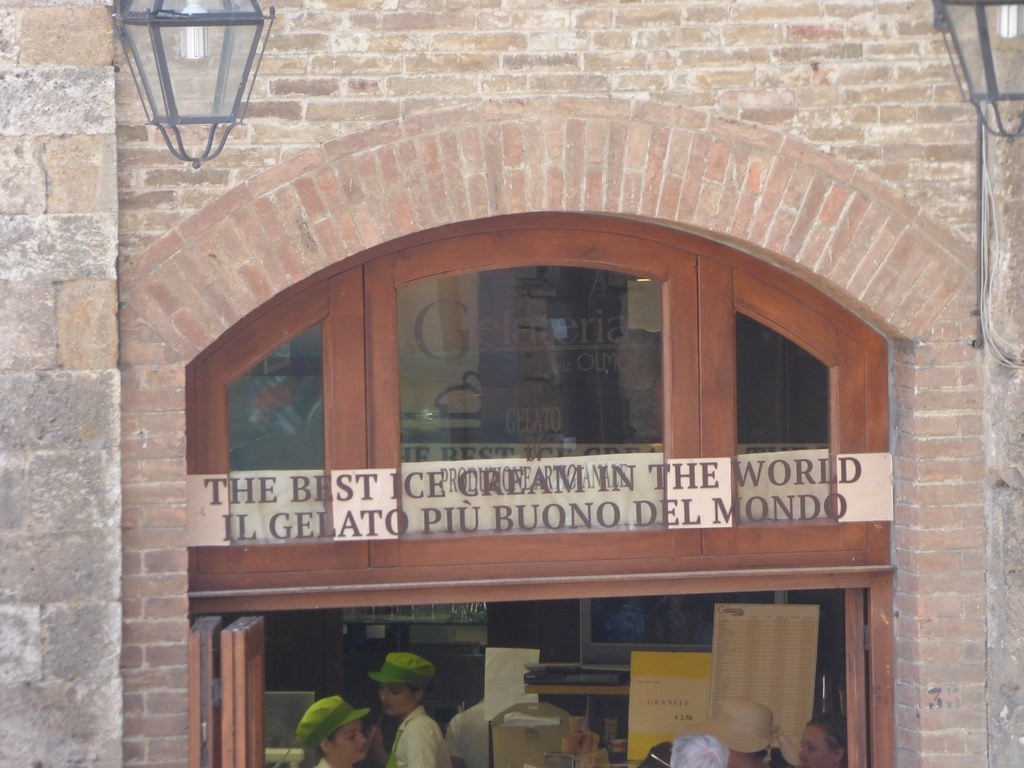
[
  {"xmin": 367, "ymin": 652, "xmax": 454, "ymax": 768},
  {"xmin": 444, "ymin": 698, "xmax": 490, "ymax": 768},
  {"xmin": 635, "ymin": 696, "xmax": 848, "ymax": 768},
  {"xmin": 296, "ymin": 695, "xmax": 370, "ymax": 768}
]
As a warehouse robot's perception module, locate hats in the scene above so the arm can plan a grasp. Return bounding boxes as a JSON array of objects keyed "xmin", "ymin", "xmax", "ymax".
[
  {"xmin": 296, "ymin": 695, "xmax": 371, "ymax": 748},
  {"xmin": 368, "ymin": 652, "xmax": 436, "ymax": 690},
  {"xmin": 688, "ymin": 698, "xmax": 773, "ymax": 754}
]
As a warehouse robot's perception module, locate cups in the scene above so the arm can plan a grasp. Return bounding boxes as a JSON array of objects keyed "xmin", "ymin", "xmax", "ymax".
[
  {"xmin": 814, "ymin": 671, "xmax": 844, "ymax": 718},
  {"xmin": 355, "ymin": 601, "xmax": 487, "ymax": 623}
]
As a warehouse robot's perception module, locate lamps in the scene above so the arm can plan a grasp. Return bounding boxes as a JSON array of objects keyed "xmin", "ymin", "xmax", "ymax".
[
  {"xmin": 933, "ymin": 0, "xmax": 1024, "ymax": 144},
  {"xmin": 113, "ymin": 0, "xmax": 276, "ymax": 168}
]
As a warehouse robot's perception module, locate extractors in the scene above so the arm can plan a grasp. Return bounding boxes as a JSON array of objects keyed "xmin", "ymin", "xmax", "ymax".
[{"xmin": 521, "ymin": 663, "xmax": 632, "ymax": 695}]
